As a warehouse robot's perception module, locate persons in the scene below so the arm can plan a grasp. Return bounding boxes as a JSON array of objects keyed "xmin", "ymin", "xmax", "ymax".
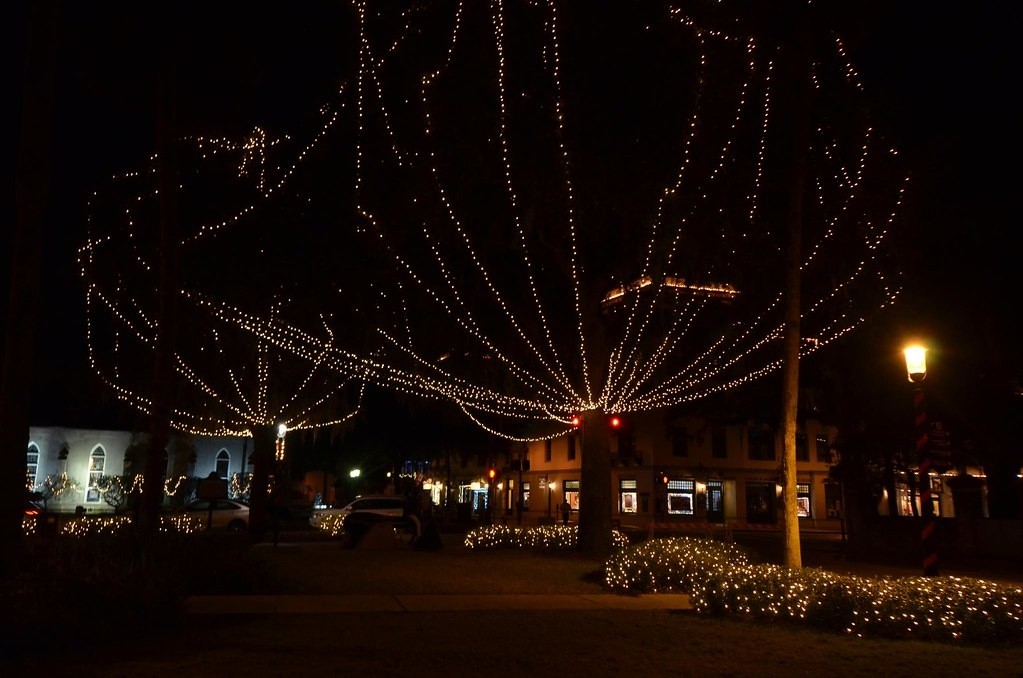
[{"xmin": 560, "ymin": 499, "xmax": 572, "ymax": 526}]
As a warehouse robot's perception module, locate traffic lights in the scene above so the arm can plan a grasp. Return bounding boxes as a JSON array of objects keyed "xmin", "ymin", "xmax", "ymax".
[{"xmin": 660, "ymin": 474, "xmax": 669, "ymax": 485}]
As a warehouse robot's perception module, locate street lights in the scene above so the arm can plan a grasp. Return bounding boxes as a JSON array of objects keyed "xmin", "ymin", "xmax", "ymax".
[
  {"xmin": 895, "ymin": 337, "xmax": 931, "ymax": 577},
  {"xmin": 489, "ymin": 469, "xmax": 495, "ymax": 520},
  {"xmin": 350, "ymin": 469, "xmax": 360, "ymax": 496}
]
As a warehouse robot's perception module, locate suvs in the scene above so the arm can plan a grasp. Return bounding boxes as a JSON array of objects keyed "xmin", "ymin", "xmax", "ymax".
[{"xmin": 311, "ymin": 497, "xmax": 406, "ymax": 531}]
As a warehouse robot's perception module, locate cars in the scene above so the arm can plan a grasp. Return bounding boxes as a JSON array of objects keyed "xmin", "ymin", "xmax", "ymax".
[
  {"xmin": 163, "ymin": 497, "xmax": 250, "ymax": 532},
  {"xmin": 26, "ymin": 501, "xmax": 55, "ymax": 525}
]
